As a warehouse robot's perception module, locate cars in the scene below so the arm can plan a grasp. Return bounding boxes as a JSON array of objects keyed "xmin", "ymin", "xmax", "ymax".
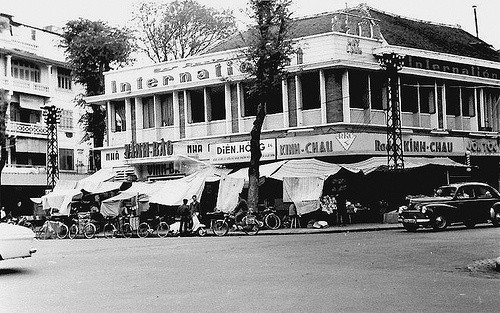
[
  {"xmin": 0, "ymin": 222, "xmax": 39, "ymax": 261},
  {"xmin": 397, "ymin": 182, "xmax": 500, "ymax": 232}
]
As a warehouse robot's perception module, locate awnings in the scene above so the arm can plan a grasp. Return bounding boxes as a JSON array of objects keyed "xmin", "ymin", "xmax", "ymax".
[{"xmin": 29, "ymin": 157, "xmax": 477, "ymax": 215}]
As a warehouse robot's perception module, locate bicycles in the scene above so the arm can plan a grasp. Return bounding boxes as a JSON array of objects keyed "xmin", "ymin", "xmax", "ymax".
[
  {"xmin": 137, "ymin": 215, "xmax": 170, "ymax": 239},
  {"xmin": 103, "ymin": 213, "xmax": 134, "ymax": 239},
  {"xmin": 38, "ymin": 216, "xmax": 69, "ymax": 239},
  {"xmin": 252, "ymin": 204, "xmax": 281, "ymax": 230},
  {"xmin": 69, "ymin": 216, "xmax": 97, "ymax": 240},
  {"xmin": 7, "ymin": 211, "xmax": 37, "ymax": 236}
]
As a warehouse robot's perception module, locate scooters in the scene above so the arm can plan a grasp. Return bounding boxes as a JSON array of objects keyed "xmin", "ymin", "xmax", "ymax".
[{"xmin": 169, "ymin": 209, "xmax": 207, "ymax": 237}]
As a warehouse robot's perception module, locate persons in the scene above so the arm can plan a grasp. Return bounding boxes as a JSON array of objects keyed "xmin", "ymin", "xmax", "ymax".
[
  {"xmin": 0, "ymin": 206, "xmax": 6, "ymax": 219},
  {"xmin": 178, "ymin": 198, "xmax": 190, "ymax": 237},
  {"xmin": 289, "ymin": 203, "xmax": 302, "ymax": 228},
  {"xmin": 119, "ymin": 204, "xmax": 129, "ymax": 231},
  {"xmin": 232, "ymin": 192, "xmax": 250, "ymax": 225},
  {"xmin": 189, "ymin": 195, "xmax": 201, "ymax": 227}
]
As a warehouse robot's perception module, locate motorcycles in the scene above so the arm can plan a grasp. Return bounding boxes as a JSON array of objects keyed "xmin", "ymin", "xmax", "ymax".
[{"xmin": 211, "ymin": 210, "xmax": 261, "ymax": 237}]
